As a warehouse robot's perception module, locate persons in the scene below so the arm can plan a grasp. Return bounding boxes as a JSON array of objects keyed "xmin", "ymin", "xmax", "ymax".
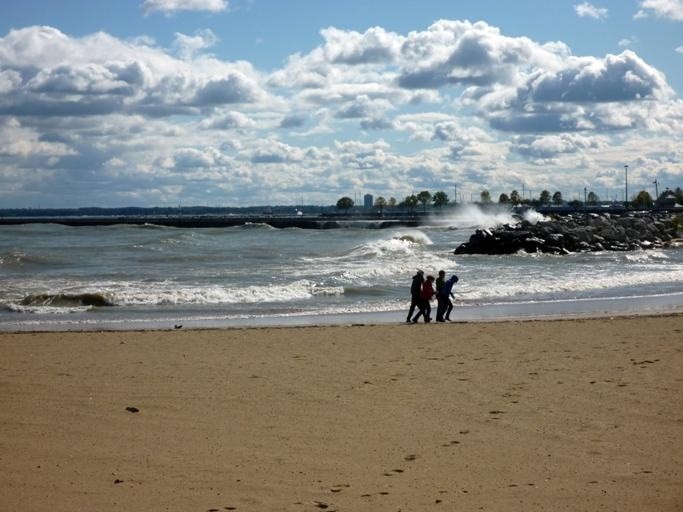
[
  {"xmin": 435, "ymin": 270, "xmax": 445, "ymax": 322},
  {"xmin": 444, "ymin": 275, "xmax": 458, "ymax": 321},
  {"xmin": 407, "ymin": 270, "xmax": 426, "ymax": 322},
  {"xmin": 413, "ymin": 275, "xmax": 435, "ymax": 322}
]
